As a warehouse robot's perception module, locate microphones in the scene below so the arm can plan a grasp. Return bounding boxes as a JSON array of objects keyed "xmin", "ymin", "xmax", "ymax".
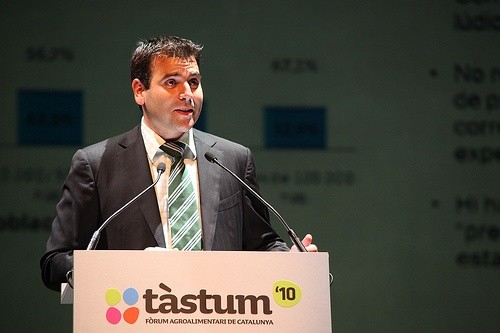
[
  {"xmin": 205, "ymin": 151, "xmax": 308, "ymax": 253},
  {"xmin": 86, "ymin": 163, "xmax": 166, "ymax": 252}
]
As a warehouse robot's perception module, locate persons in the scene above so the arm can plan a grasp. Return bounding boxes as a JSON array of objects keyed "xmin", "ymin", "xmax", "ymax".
[{"xmin": 40, "ymin": 34, "xmax": 318, "ymax": 291}]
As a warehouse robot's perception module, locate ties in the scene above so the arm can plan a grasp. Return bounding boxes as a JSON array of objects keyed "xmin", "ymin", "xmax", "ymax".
[{"xmin": 159, "ymin": 142, "xmax": 202, "ymax": 251}]
